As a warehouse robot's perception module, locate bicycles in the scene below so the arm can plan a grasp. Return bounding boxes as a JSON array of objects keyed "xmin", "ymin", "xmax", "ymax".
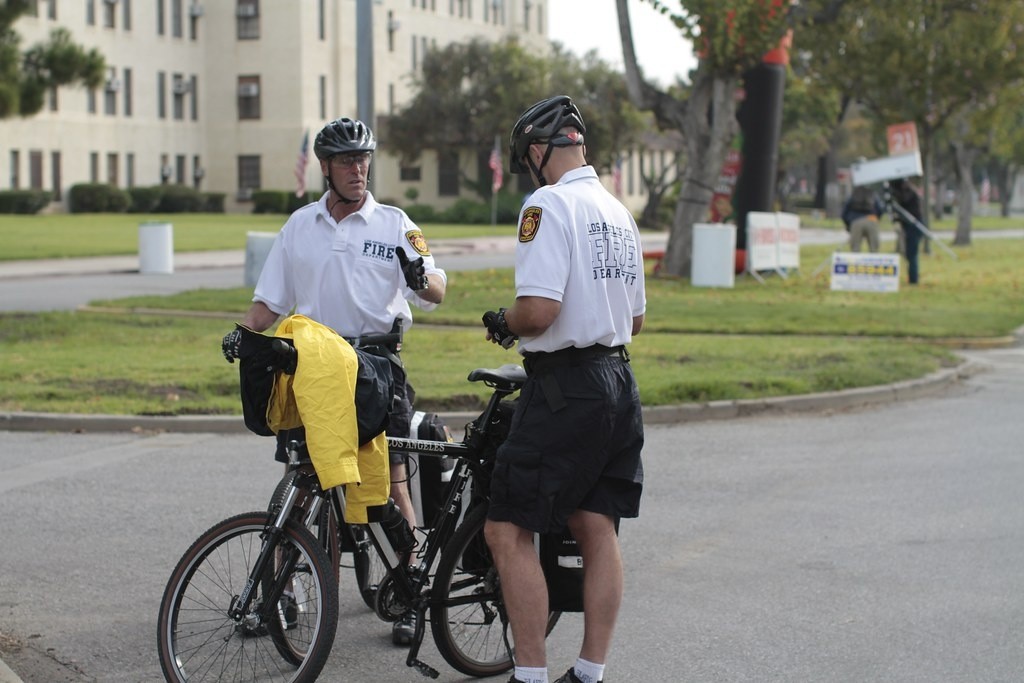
[{"xmin": 155, "ymin": 343, "xmax": 570, "ymax": 683}]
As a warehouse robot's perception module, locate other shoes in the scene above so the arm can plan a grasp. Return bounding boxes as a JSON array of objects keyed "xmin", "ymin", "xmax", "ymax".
[
  {"xmin": 505, "ymin": 674, "xmax": 525, "ymax": 683},
  {"xmin": 553, "ymin": 667, "xmax": 601, "ymax": 683}
]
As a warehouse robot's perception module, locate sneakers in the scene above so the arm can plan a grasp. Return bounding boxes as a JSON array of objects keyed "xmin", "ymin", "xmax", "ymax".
[
  {"xmin": 237, "ymin": 592, "xmax": 298, "ymax": 634},
  {"xmin": 393, "ymin": 610, "xmax": 416, "ymax": 645}
]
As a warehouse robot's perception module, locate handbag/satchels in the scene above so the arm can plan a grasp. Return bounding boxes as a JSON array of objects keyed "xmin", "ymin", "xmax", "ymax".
[
  {"xmin": 536, "ymin": 532, "xmax": 585, "ymax": 612},
  {"xmin": 446, "ymin": 460, "xmax": 485, "ymax": 573},
  {"xmin": 408, "ymin": 411, "xmax": 455, "ymax": 529}
]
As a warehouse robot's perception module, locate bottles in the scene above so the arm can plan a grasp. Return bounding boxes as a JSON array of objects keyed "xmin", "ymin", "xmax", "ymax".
[{"xmin": 380, "ymin": 499, "xmax": 415, "ymax": 552}]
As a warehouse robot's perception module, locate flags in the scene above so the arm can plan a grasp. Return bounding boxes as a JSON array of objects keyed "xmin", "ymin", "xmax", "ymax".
[
  {"xmin": 292, "ymin": 130, "xmax": 310, "ymax": 199},
  {"xmin": 489, "ymin": 137, "xmax": 503, "ymax": 193}
]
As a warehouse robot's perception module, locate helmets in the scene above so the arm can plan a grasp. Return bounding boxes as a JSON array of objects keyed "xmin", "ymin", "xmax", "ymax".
[
  {"xmin": 314, "ymin": 118, "xmax": 376, "ymax": 160},
  {"xmin": 509, "ymin": 95, "xmax": 586, "ymax": 174}
]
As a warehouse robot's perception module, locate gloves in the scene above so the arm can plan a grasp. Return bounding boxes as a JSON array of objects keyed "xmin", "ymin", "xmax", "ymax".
[
  {"xmin": 395, "ymin": 246, "xmax": 429, "ymax": 290},
  {"xmin": 222, "ymin": 322, "xmax": 250, "ymax": 363}
]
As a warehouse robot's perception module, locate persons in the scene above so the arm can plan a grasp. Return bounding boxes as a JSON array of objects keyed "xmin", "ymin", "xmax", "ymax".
[
  {"xmin": 894, "ymin": 178, "xmax": 923, "ymax": 283},
  {"xmin": 223, "ymin": 117, "xmax": 448, "ymax": 646},
  {"xmin": 483, "ymin": 95, "xmax": 647, "ymax": 683},
  {"xmin": 842, "ymin": 185, "xmax": 888, "ymax": 254}
]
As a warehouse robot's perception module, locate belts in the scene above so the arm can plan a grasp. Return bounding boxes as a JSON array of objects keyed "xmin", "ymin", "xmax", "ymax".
[{"xmin": 523, "ymin": 342, "xmax": 623, "ymax": 374}]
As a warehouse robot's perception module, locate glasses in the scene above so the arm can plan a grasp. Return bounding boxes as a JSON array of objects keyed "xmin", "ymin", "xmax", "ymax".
[{"xmin": 332, "ymin": 153, "xmax": 371, "ymax": 167}]
